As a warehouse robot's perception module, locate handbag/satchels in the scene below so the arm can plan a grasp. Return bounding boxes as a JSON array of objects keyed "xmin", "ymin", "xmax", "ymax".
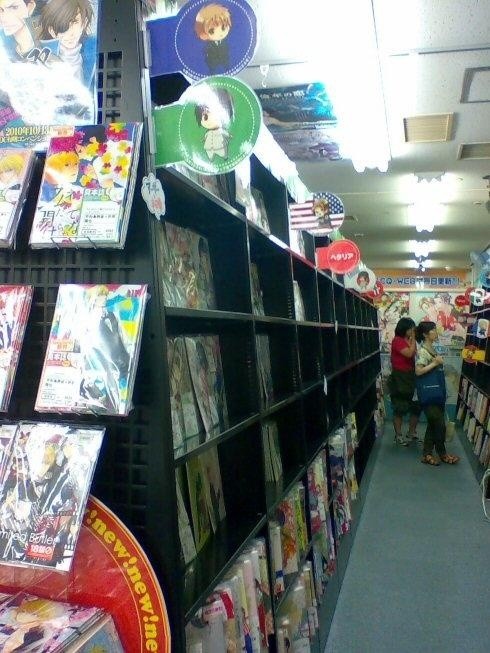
[
  {"xmin": 414, "ymin": 345, "xmax": 447, "ymax": 407},
  {"xmin": 443, "ymin": 409, "xmax": 456, "ymax": 443}
]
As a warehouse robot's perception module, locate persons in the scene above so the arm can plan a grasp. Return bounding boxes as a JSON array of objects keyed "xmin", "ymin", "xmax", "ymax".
[
  {"xmin": 388, "ymin": 316, "xmax": 424, "ymax": 446},
  {"xmin": 413, "ymin": 320, "xmax": 462, "ymax": 466}
]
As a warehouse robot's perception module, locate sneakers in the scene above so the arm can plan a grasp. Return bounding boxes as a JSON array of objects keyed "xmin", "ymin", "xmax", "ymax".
[
  {"xmin": 406, "ymin": 431, "xmax": 420, "ymax": 441},
  {"xmin": 393, "ymin": 434, "xmax": 408, "ymax": 447}
]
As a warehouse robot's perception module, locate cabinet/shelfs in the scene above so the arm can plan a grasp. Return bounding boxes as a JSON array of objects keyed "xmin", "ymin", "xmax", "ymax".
[
  {"xmin": 451, "ymin": 293, "xmax": 490, "ymax": 496},
  {"xmin": 0, "ymin": 0, "xmax": 381, "ymax": 653}
]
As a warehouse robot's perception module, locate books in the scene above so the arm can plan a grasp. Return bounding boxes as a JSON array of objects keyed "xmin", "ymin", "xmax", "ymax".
[
  {"xmin": 291, "ymin": 228, "xmax": 307, "ymax": 259},
  {"xmin": 1, "ymin": 1, "xmax": 99, "ymax": 152},
  {"xmin": 177, "ymin": 445, "xmax": 228, "ymax": 564},
  {"xmin": 1, "ymin": 147, "xmax": 36, "ymax": 248},
  {"xmin": 2, "ymin": 421, "xmax": 105, "ymax": 572},
  {"xmin": 292, "ymin": 280, "xmax": 305, "ymax": 321},
  {"xmin": 155, "ymin": 220, "xmax": 217, "ymax": 310},
  {"xmin": 172, "ymin": 160, "xmax": 272, "ymax": 233},
  {"xmin": 29, "ymin": 121, "xmax": 144, "ymax": 250},
  {"xmin": 455, "ymin": 378, "xmax": 490, "ymax": 472},
  {"xmin": 1, "ymin": 285, "xmax": 36, "ymax": 414},
  {"xmin": 34, "ymin": 284, "xmax": 152, "ymax": 416},
  {"xmin": 184, "ymin": 411, "xmax": 358, "ymax": 652},
  {"xmin": 165, "ymin": 334, "xmax": 228, "ymax": 450},
  {"xmin": 2, "ymin": 591, "xmax": 124, "ymax": 652},
  {"xmin": 248, "ymin": 261, "xmax": 284, "ymax": 481}
]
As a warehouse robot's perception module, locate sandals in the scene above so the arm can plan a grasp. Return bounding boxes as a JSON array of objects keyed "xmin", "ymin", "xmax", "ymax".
[
  {"xmin": 421, "ymin": 455, "xmax": 440, "ymax": 465},
  {"xmin": 440, "ymin": 453, "xmax": 460, "ymax": 465}
]
